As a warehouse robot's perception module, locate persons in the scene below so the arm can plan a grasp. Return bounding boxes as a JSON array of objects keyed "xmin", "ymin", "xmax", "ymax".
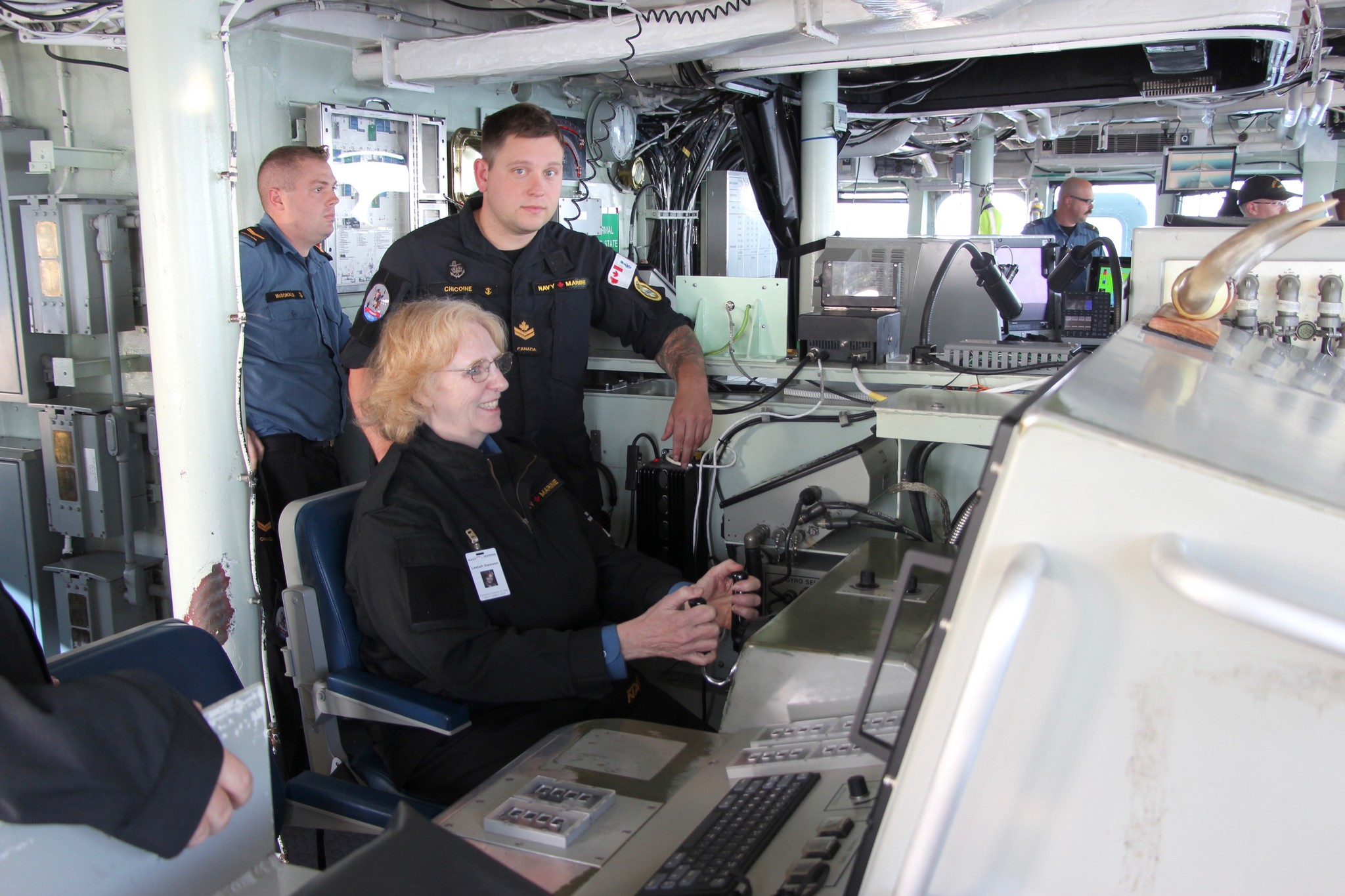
[
  {"xmin": 342, "ymin": 300, "xmax": 762, "ymax": 817},
  {"xmin": 1237, "ymin": 174, "xmax": 1303, "ymax": 219},
  {"xmin": 0, "ymin": 568, "xmax": 252, "ymax": 858},
  {"xmin": 344, "ymin": 104, "xmax": 713, "ymax": 539},
  {"xmin": 1021, "ymin": 177, "xmax": 1106, "ymax": 293},
  {"xmin": 238, "ymin": 147, "xmax": 351, "ymax": 778}
]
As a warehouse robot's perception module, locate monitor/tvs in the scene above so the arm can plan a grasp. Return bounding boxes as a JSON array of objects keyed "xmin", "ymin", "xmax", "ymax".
[
  {"xmin": 994, "ymin": 238, "xmax": 1054, "ymax": 331},
  {"xmin": 1160, "ymin": 145, "xmax": 1239, "ymax": 196},
  {"xmin": 1087, "ymin": 256, "xmax": 1131, "ymax": 324}
]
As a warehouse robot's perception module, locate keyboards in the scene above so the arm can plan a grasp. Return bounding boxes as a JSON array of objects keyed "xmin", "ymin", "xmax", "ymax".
[{"xmin": 634, "ymin": 772, "xmax": 821, "ymax": 896}]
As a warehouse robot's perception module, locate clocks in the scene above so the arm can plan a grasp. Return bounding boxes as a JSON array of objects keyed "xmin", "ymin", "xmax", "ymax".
[{"xmin": 586, "ymin": 93, "xmax": 636, "ymax": 167}]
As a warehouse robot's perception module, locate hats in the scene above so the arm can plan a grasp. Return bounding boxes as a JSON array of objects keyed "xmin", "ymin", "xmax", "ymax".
[{"xmin": 1236, "ymin": 174, "xmax": 1303, "ymax": 205}]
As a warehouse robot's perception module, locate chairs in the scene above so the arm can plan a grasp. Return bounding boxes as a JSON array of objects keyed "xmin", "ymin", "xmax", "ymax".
[{"xmin": 0, "ymin": 481, "xmax": 474, "ymax": 896}]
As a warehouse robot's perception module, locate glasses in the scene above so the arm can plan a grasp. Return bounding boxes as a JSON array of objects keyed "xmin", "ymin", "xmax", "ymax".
[
  {"xmin": 435, "ymin": 351, "xmax": 513, "ymax": 383},
  {"xmin": 1253, "ymin": 199, "xmax": 1290, "ymax": 208},
  {"xmin": 1070, "ymin": 195, "xmax": 1094, "ymax": 205}
]
